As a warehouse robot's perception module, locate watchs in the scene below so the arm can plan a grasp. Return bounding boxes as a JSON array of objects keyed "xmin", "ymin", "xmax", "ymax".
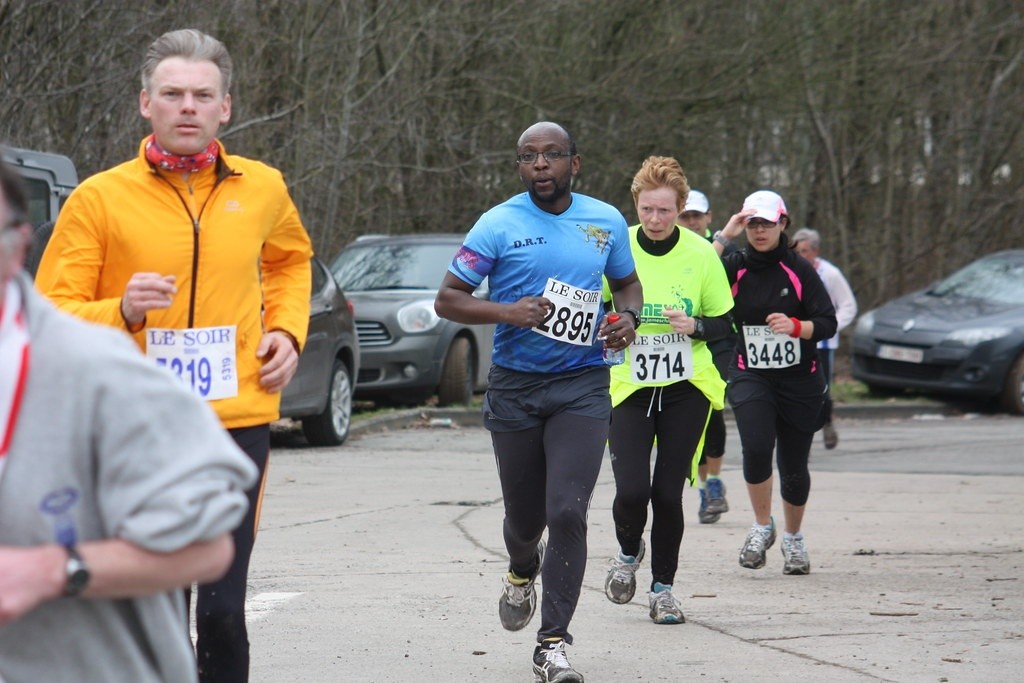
[
  {"xmin": 687, "ymin": 318, "xmax": 704, "ymax": 339},
  {"xmin": 60, "ymin": 542, "xmax": 92, "ymax": 598}
]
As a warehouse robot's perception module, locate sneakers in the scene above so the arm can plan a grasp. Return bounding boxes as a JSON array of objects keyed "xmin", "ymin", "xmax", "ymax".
[
  {"xmin": 499, "ymin": 538, "xmax": 547, "ymax": 632},
  {"xmin": 705, "ymin": 477, "xmax": 727, "ymax": 513},
  {"xmin": 605, "ymin": 539, "xmax": 645, "ymax": 604},
  {"xmin": 698, "ymin": 487, "xmax": 720, "ymax": 523},
  {"xmin": 533, "ymin": 639, "xmax": 584, "ymax": 683},
  {"xmin": 780, "ymin": 533, "xmax": 810, "ymax": 575},
  {"xmin": 739, "ymin": 516, "xmax": 777, "ymax": 569},
  {"xmin": 649, "ymin": 582, "xmax": 685, "ymax": 624}
]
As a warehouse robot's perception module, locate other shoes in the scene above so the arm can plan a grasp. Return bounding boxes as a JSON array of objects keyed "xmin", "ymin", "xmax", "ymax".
[{"xmin": 822, "ymin": 423, "xmax": 837, "ymax": 449}]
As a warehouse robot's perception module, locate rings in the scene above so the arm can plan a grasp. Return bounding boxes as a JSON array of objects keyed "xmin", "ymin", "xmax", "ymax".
[{"xmin": 623, "ymin": 337, "xmax": 627, "ymax": 343}]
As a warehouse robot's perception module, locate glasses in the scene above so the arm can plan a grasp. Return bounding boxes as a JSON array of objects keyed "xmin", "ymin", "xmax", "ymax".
[
  {"xmin": 515, "ymin": 150, "xmax": 576, "ymax": 165},
  {"xmin": 746, "ymin": 219, "xmax": 783, "ymax": 230}
]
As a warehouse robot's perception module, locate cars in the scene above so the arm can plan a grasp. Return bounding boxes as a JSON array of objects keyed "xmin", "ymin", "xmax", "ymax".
[
  {"xmin": 256, "ymin": 253, "xmax": 359, "ymax": 450},
  {"xmin": 847, "ymin": 249, "xmax": 1024, "ymax": 418},
  {"xmin": 322, "ymin": 232, "xmax": 494, "ymax": 407}
]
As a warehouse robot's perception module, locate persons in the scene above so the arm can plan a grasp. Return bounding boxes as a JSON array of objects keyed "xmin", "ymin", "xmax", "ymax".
[
  {"xmin": 33, "ymin": 28, "xmax": 314, "ymax": 683},
  {"xmin": 792, "ymin": 229, "xmax": 859, "ymax": 450},
  {"xmin": 676, "ymin": 189, "xmax": 728, "ymax": 523},
  {"xmin": 1, "ymin": 142, "xmax": 259, "ymax": 682},
  {"xmin": 600, "ymin": 157, "xmax": 735, "ymax": 622},
  {"xmin": 710, "ymin": 189, "xmax": 837, "ymax": 578},
  {"xmin": 434, "ymin": 121, "xmax": 644, "ymax": 683}
]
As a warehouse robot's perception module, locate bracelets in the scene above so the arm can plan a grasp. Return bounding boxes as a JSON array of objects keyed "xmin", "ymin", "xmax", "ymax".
[
  {"xmin": 712, "ymin": 231, "xmax": 731, "ymax": 248},
  {"xmin": 789, "ymin": 317, "xmax": 802, "ymax": 339},
  {"xmin": 623, "ymin": 308, "xmax": 641, "ymax": 330}
]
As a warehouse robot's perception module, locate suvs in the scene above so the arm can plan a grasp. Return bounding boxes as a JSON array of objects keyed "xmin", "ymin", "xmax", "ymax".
[{"xmin": 0, "ymin": 143, "xmax": 79, "ymax": 283}]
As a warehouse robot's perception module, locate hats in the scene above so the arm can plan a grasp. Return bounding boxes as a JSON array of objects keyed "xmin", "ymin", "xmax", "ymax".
[
  {"xmin": 678, "ymin": 190, "xmax": 709, "ymax": 216},
  {"xmin": 741, "ymin": 190, "xmax": 787, "ymax": 226}
]
